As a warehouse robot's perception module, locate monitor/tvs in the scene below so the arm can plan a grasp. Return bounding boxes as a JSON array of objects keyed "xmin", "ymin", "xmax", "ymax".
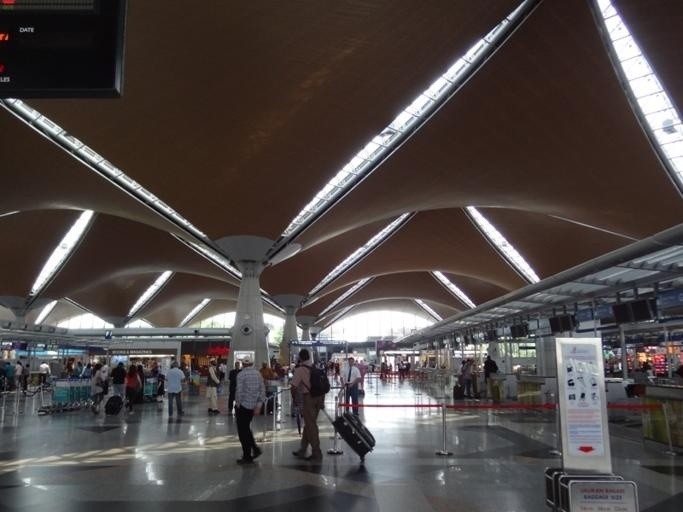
[
  {"xmin": 612, "ymin": 302, "xmax": 636, "ymax": 326},
  {"xmin": 428, "ymin": 322, "xmax": 527, "ymax": 349},
  {"xmin": 560, "ymin": 314, "xmax": 576, "ymax": 332},
  {"xmin": 549, "ymin": 316, "xmax": 564, "ymax": 333},
  {"xmin": 631, "ymin": 298, "xmax": 657, "ymax": 324}
]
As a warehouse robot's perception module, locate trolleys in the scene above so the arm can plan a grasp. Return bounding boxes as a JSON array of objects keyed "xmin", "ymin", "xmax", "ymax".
[
  {"xmin": 36, "ymin": 379, "xmax": 92, "ymax": 416},
  {"xmin": 143, "ymin": 372, "xmax": 160, "ymax": 404}
]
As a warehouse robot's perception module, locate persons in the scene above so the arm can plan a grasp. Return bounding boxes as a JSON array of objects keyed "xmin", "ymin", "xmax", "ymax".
[
  {"xmin": 125, "ymin": 364, "xmax": 143, "ymax": 412},
  {"xmin": 157, "ymin": 369, "xmax": 165, "ymax": 403},
  {"xmin": 228, "ymin": 361, "xmax": 242, "ymax": 415},
  {"xmin": 460, "ymin": 355, "xmax": 498, "ymax": 399},
  {"xmin": 289, "ymin": 351, "xmax": 326, "ymax": 461},
  {"xmin": 340, "ymin": 357, "xmax": 362, "ymax": 416},
  {"xmin": 290, "ymin": 360, "xmax": 305, "ymax": 419},
  {"xmin": 110, "ymin": 362, "xmax": 127, "ymax": 399},
  {"xmin": 39, "ymin": 361, "xmax": 52, "ymax": 384},
  {"xmin": 399, "ymin": 361, "xmax": 405, "ymax": 380},
  {"xmin": 234, "ymin": 357, "xmax": 267, "ymax": 462},
  {"xmin": 136, "ymin": 365, "xmax": 145, "ymax": 405},
  {"xmin": 13, "ymin": 360, "xmax": 22, "ymax": 388},
  {"xmin": 100, "ymin": 361, "xmax": 109, "ymax": 395},
  {"xmin": 90, "ymin": 364, "xmax": 105, "ymax": 411},
  {"xmin": 167, "ymin": 362, "xmax": 186, "ymax": 416},
  {"xmin": 151, "ymin": 362, "xmax": 159, "ymax": 378},
  {"xmin": 59, "ymin": 361, "xmax": 93, "ymax": 379},
  {"xmin": 208, "ymin": 358, "xmax": 221, "ymax": 414},
  {"xmin": 260, "ymin": 362, "xmax": 277, "ymax": 415},
  {"xmin": 3, "ymin": 362, "xmax": 16, "ymax": 392},
  {"xmin": 381, "ymin": 362, "xmax": 388, "ymax": 378},
  {"xmin": 22, "ymin": 364, "xmax": 32, "ymax": 385},
  {"xmin": 318, "ymin": 358, "xmax": 341, "ymax": 376}
]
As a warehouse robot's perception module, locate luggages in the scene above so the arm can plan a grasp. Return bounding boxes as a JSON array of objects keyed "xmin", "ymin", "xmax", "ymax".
[{"xmin": 321, "ymin": 404, "xmax": 375, "ymax": 461}]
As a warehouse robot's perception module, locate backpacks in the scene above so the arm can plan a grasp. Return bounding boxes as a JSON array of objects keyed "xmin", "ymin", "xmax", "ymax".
[{"xmin": 295, "ymin": 364, "xmax": 329, "ymax": 394}]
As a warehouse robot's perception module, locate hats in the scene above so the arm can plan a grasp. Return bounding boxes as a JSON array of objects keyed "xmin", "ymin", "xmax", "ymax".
[{"xmin": 242, "ymin": 356, "xmax": 253, "ymax": 364}]
